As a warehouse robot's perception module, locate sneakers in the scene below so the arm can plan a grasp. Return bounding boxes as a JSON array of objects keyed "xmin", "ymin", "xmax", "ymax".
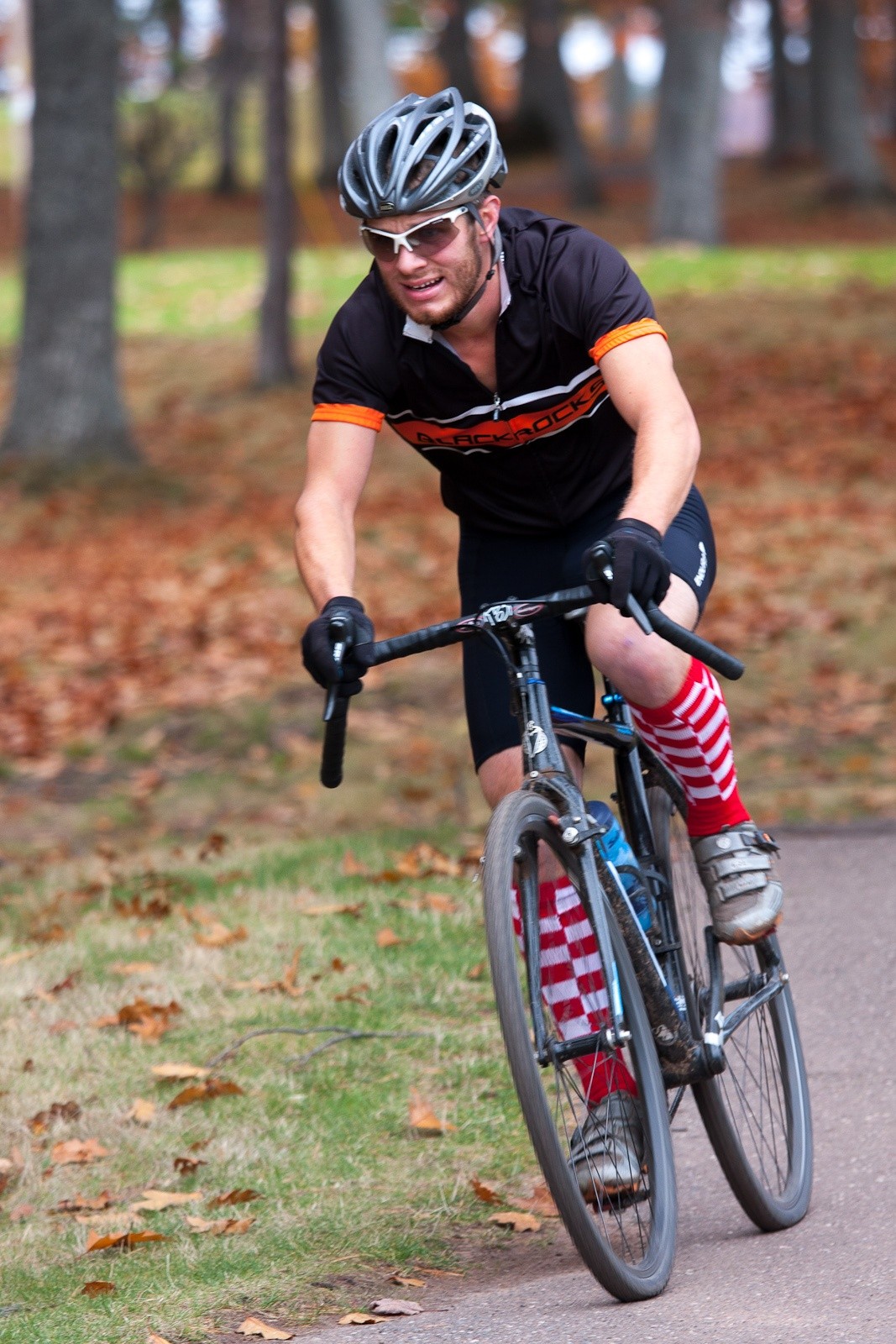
[
  {"xmin": 688, "ymin": 819, "xmax": 782, "ymax": 944},
  {"xmin": 566, "ymin": 1090, "xmax": 648, "ymax": 1193}
]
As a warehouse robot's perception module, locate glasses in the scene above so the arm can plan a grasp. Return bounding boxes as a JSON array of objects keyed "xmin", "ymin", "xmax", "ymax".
[{"xmin": 359, "ymin": 207, "xmax": 469, "ymax": 262}]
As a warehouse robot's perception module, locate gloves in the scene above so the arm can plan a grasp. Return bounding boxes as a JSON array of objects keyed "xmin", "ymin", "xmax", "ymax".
[
  {"xmin": 300, "ymin": 596, "xmax": 374, "ymax": 694},
  {"xmin": 585, "ymin": 518, "xmax": 671, "ymax": 618}
]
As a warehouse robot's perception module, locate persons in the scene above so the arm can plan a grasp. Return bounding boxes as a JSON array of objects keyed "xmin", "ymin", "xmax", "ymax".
[{"xmin": 294, "ymin": 87, "xmax": 782, "ymax": 1211}]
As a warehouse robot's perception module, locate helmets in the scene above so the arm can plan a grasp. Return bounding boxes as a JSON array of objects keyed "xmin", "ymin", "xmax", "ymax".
[{"xmin": 337, "ymin": 87, "xmax": 508, "ymax": 219}]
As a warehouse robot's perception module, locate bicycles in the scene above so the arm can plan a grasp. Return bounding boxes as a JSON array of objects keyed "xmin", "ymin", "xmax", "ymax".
[{"xmin": 319, "ymin": 542, "xmax": 814, "ymax": 1301}]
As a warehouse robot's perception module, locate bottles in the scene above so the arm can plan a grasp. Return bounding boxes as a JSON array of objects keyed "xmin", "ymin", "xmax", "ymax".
[{"xmin": 583, "ymin": 794, "xmax": 654, "ymax": 933}]
